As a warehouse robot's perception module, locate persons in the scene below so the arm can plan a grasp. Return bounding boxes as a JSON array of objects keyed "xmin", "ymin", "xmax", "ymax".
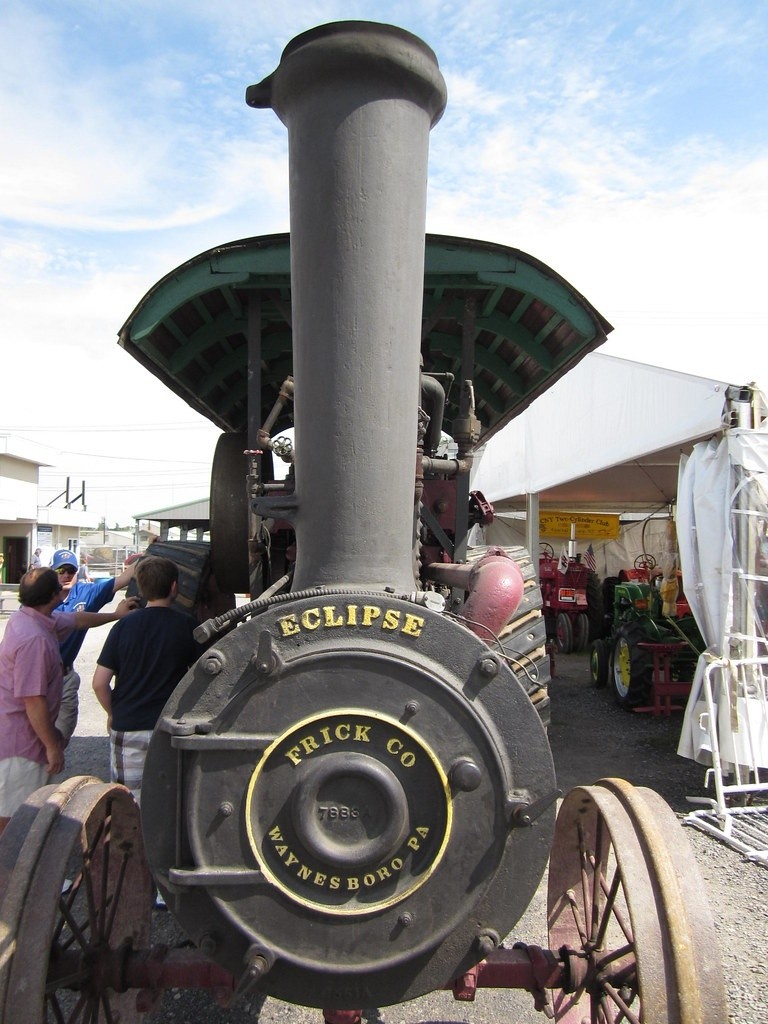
[
  {"xmin": 92, "ymin": 557, "xmax": 207, "ymax": 907},
  {"xmin": 0, "ymin": 567, "xmax": 141, "ymax": 893},
  {"xmin": 30, "ymin": 547, "xmax": 42, "ymax": 569},
  {"xmin": 47, "ymin": 550, "xmax": 140, "ymax": 747},
  {"xmin": 79, "ymin": 559, "xmax": 90, "ymax": 582}
]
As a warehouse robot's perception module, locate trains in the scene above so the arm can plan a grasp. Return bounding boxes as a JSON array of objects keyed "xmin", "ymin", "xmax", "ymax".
[{"xmin": 0, "ymin": 17, "xmax": 724, "ymax": 1024}]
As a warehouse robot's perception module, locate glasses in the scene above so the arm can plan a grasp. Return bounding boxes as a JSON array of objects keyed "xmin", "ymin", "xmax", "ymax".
[
  {"xmin": 56, "ymin": 584, "xmax": 62, "ymax": 592},
  {"xmin": 55, "ymin": 567, "xmax": 77, "ymax": 574}
]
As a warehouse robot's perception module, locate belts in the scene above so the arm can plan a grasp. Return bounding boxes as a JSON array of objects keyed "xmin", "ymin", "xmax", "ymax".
[{"xmin": 63, "ymin": 664, "xmax": 73, "ymax": 674}]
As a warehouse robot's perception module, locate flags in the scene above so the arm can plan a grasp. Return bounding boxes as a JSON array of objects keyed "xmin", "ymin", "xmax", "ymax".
[
  {"xmin": 584, "ymin": 544, "xmax": 596, "ymax": 571},
  {"xmin": 557, "ymin": 545, "xmax": 569, "ymax": 575}
]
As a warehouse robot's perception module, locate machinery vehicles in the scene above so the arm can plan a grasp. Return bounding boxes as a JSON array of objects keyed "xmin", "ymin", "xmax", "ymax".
[{"xmin": 534, "ymin": 539, "xmax": 706, "ymax": 720}]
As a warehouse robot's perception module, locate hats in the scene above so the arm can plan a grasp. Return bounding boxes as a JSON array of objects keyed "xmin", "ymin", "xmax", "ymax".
[{"xmin": 51, "ymin": 550, "xmax": 78, "ymax": 571}]
